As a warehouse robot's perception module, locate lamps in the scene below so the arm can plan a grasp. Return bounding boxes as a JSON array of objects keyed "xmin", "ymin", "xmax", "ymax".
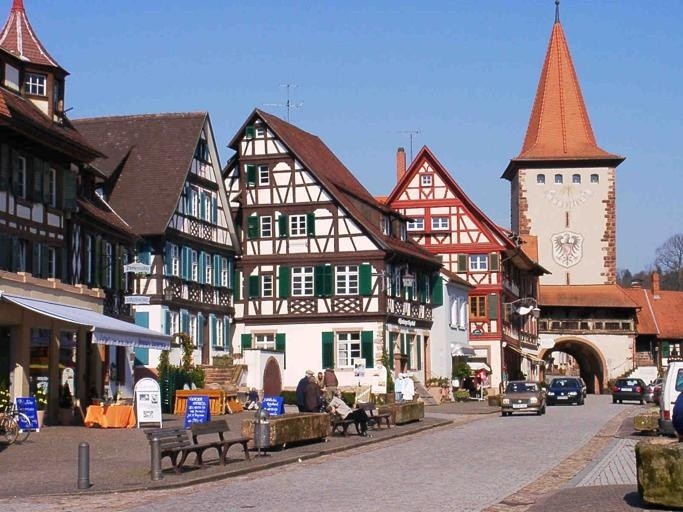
[
  {"xmin": 386, "ymin": 261, "xmax": 415, "ymax": 289},
  {"xmin": 504, "ymin": 297, "xmax": 540, "ymax": 318},
  {"xmin": 161, "ymin": 286, "xmax": 175, "ymax": 302}
]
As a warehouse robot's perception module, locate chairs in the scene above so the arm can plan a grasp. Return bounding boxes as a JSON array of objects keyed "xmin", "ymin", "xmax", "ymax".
[{"xmin": 622, "ymin": 381, "xmax": 628, "ymax": 386}]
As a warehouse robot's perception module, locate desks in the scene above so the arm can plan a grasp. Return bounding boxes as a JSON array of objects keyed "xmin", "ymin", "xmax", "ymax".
[{"xmin": 84, "ymin": 401, "xmax": 137, "ymax": 429}]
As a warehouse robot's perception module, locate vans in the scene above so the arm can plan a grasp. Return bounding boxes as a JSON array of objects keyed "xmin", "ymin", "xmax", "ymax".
[{"xmin": 658, "ymin": 361, "xmax": 683, "ymax": 435}]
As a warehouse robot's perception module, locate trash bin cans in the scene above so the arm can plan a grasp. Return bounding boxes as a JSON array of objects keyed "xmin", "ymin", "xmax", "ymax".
[{"xmin": 253, "ymin": 406, "xmax": 271, "ymax": 455}]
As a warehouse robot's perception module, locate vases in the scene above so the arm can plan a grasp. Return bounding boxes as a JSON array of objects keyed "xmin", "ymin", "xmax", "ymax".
[
  {"xmin": 0, "ymin": 412, "xmax": 6, "ymax": 421},
  {"xmin": 31, "ymin": 411, "xmax": 43, "ymax": 429}
]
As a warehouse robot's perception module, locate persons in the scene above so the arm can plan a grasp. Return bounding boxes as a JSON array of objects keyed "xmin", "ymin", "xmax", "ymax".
[
  {"xmin": 297, "ymin": 367, "xmax": 374, "ymax": 437},
  {"xmin": 461, "ymin": 377, "xmax": 475, "ymax": 396},
  {"xmin": 246, "ymin": 387, "xmax": 260, "ymax": 410}
]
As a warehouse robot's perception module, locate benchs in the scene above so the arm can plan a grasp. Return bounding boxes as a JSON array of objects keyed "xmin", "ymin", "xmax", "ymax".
[
  {"xmin": 328, "ymin": 410, "xmax": 366, "ymax": 438},
  {"xmin": 136, "ymin": 422, "xmax": 220, "ymax": 475},
  {"xmin": 189, "ymin": 420, "xmax": 252, "ymax": 468},
  {"xmin": 356, "ymin": 402, "xmax": 391, "ymax": 430}
]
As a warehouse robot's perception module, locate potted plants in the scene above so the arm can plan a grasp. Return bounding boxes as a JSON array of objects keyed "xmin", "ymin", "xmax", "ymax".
[
  {"xmin": 453, "ymin": 391, "xmax": 469, "ymax": 402},
  {"xmin": 60, "ymin": 383, "xmax": 73, "ymax": 426}
]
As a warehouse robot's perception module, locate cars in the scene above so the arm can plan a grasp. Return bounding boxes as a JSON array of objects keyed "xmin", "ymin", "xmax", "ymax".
[
  {"xmin": 611, "ymin": 377, "xmax": 648, "ymax": 405},
  {"xmin": 576, "ymin": 377, "xmax": 586, "ymax": 397},
  {"xmin": 545, "ymin": 377, "xmax": 584, "ymax": 405},
  {"xmin": 645, "ymin": 377, "xmax": 663, "ymax": 403},
  {"xmin": 500, "ymin": 380, "xmax": 546, "ymax": 416},
  {"xmin": 651, "ymin": 382, "xmax": 661, "ymax": 403}
]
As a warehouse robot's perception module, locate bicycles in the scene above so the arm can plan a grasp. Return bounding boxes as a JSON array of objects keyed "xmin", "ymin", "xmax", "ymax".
[{"xmin": 0, "ymin": 399, "xmax": 31, "ymax": 446}]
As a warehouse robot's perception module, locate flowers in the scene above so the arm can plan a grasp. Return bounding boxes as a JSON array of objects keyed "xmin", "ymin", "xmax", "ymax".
[
  {"xmin": 0, "ymin": 377, "xmax": 14, "ymax": 419},
  {"xmin": 25, "ymin": 389, "xmax": 46, "ymax": 410}
]
